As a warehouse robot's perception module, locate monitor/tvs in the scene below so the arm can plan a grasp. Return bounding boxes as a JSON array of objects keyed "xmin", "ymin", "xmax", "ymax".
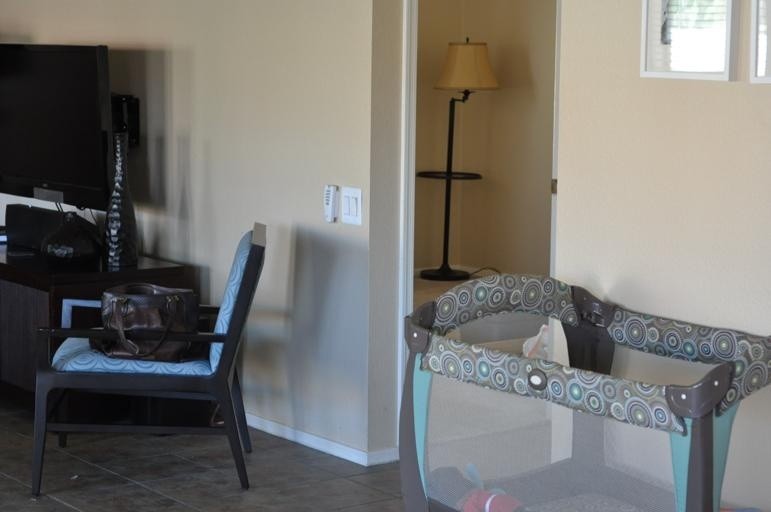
[{"xmin": 0, "ymin": 43, "xmax": 115, "ymax": 211}]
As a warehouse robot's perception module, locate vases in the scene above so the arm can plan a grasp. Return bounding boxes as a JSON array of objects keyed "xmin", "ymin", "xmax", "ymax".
[{"xmin": 104, "ymin": 130, "xmax": 140, "ymax": 269}]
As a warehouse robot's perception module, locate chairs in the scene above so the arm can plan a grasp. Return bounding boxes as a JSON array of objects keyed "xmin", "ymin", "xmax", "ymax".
[{"xmin": 29, "ymin": 222, "xmax": 269, "ymax": 498}]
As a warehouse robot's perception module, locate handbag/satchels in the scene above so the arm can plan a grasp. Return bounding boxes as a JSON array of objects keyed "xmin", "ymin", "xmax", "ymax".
[{"xmin": 88, "ymin": 281, "xmax": 201, "ymax": 363}]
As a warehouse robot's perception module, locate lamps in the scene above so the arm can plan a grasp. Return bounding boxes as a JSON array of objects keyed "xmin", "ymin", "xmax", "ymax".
[{"xmin": 414, "ymin": 36, "xmax": 504, "ymax": 281}]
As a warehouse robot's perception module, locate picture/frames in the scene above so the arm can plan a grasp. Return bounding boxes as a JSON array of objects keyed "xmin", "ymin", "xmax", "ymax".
[{"xmin": 638, "ymin": 0, "xmax": 771, "ymax": 84}]
